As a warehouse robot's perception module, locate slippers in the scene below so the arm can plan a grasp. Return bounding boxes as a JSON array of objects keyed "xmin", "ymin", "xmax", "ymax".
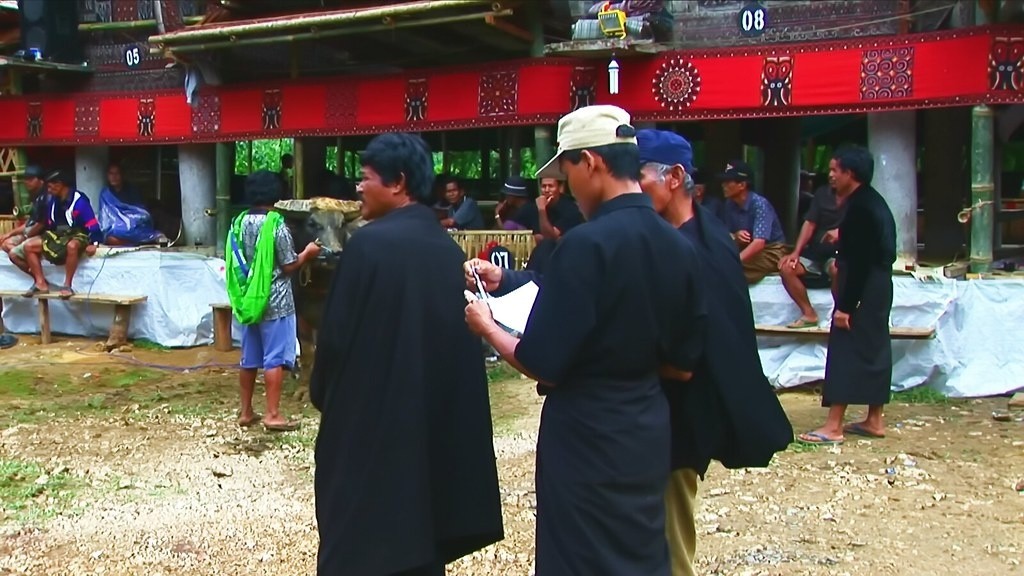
[
  {"xmin": 238, "ymin": 412, "xmax": 260, "ymax": 425},
  {"xmin": 796, "ymin": 431, "xmax": 844, "ymax": 445},
  {"xmin": 789, "ymin": 319, "xmax": 817, "ymax": 328},
  {"xmin": 267, "ymin": 419, "xmax": 301, "ymax": 430},
  {"xmin": 58, "ymin": 287, "xmax": 75, "ymax": 297},
  {"xmin": 22, "ymin": 284, "xmax": 49, "ymax": 297},
  {"xmin": 843, "ymin": 423, "xmax": 885, "ymax": 439}
]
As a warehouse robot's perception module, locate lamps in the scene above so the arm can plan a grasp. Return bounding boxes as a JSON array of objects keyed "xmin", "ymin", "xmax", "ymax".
[{"xmin": 597, "ymin": 10, "xmax": 627, "ymax": 40}]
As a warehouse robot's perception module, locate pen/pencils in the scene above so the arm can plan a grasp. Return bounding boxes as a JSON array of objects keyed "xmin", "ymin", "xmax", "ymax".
[{"xmin": 472, "ymin": 267, "xmax": 488, "ymax": 300}]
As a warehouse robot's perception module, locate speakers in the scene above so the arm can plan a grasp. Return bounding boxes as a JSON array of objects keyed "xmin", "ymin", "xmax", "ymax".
[{"xmin": 18, "ymin": 0, "xmax": 78, "ymax": 64}]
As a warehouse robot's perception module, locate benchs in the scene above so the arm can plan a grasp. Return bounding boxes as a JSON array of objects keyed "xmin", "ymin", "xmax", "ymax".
[{"xmin": 0, "ymin": 291, "xmax": 149, "ymax": 350}]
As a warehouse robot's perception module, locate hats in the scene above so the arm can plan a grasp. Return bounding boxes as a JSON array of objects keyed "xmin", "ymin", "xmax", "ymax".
[
  {"xmin": 500, "ymin": 176, "xmax": 530, "ymax": 198},
  {"xmin": 16, "ymin": 165, "xmax": 47, "ymax": 180},
  {"xmin": 635, "ymin": 129, "xmax": 692, "ymax": 172},
  {"xmin": 535, "ymin": 105, "xmax": 637, "ymax": 181},
  {"xmin": 43, "ymin": 169, "xmax": 63, "ymax": 182},
  {"xmin": 691, "ymin": 172, "xmax": 705, "ymax": 185},
  {"xmin": 715, "ymin": 160, "xmax": 751, "ymax": 181}
]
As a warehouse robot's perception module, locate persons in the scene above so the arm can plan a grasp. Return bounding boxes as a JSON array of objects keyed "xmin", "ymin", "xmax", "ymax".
[
  {"xmin": 225, "ymin": 170, "xmax": 323, "ymax": 433},
  {"xmin": 433, "ymin": 105, "xmax": 897, "ymax": 575},
  {"xmin": 278, "ymin": 154, "xmax": 294, "ymax": 201},
  {"xmin": 310, "ymin": 130, "xmax": 507, "ymax": 576},
  {"xmin": 0, "ymin": 163, "xmax": 155, "ymax": 298}
]
